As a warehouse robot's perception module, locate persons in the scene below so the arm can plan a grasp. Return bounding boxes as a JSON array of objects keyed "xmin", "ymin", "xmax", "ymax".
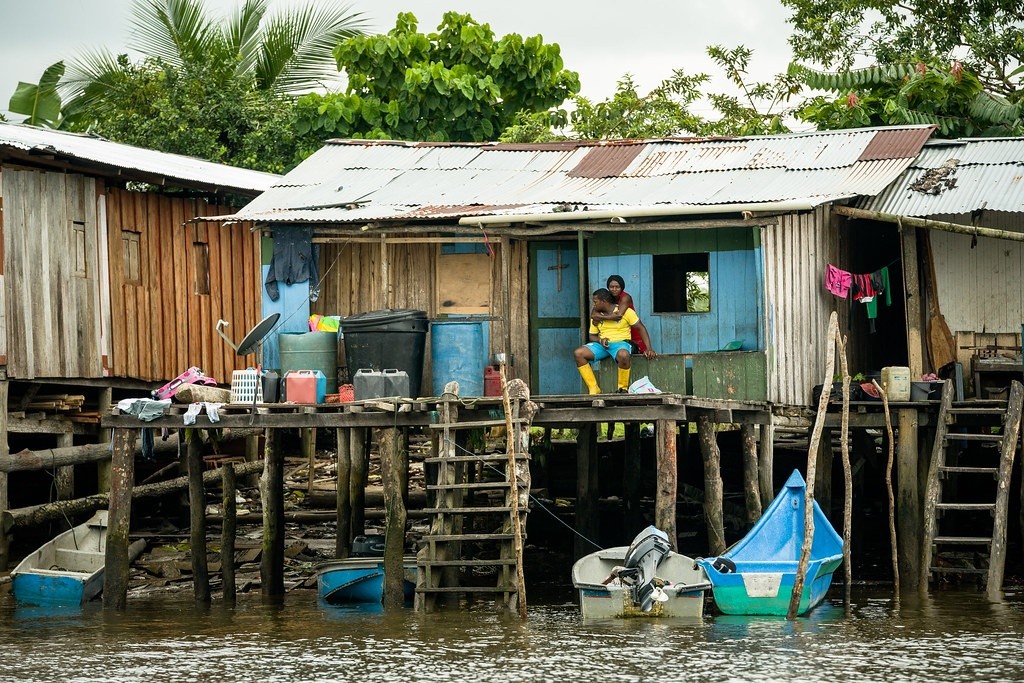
[
  {"xmin": 591, "ymin": 275, "xmax": 656, "ymax": 360},
  {"xmin": 574, "ymin": 288, "xmax": 657, "ymax": 397}
]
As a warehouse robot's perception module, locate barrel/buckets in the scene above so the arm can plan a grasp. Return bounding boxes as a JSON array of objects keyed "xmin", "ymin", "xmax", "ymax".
[
  {"xmin": 353, "ymin": 368, "xmax": 381, "ymax": 402},
  {"xmin": 279, "ymin": 330, "xmax": 339, "ymax": 395},
  {"xmin": 882, "ymin": 366, "xmax": 911, "ymax": 401},
  {"xmin": 432, "ymin": 321, "xmax": 484, "ymax": 397},
  {"xmin": 910, "ymin": 380, "xmax": 930, "ymax": 400},
  {"xmin": 380, "ymin": 368, "xmax": 409, "ymax": 398},
  {"xmin": 242, "ymin": 366, "xmax": 327, "ymax": 404},
  {"xmin": 486, "ymin": 364, "xmax": 503, "ymax": 396},
  {"xmin": 339, "ymin": 309, "xmax": 431, "ymax": 400}
]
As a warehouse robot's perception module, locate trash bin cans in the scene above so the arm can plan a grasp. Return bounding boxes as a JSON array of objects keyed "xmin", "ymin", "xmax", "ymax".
[{"xmin": 338, "ymin": 308, "xmax": 429, "ymax": 400}]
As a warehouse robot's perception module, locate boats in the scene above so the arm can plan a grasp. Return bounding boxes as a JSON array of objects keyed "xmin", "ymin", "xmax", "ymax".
[
  {"xmin": 696, "ymin": 469, "xmax": 851, "ymax": 615},
  {"xmin": 10, "ymin": 509, "xmax": 108, "ymax": 607},
  {"xmin": 572, "ymin": 526, "xmax": 710, "ymax": 619},
  {"xmin": 319, "ymin": 557, "xmax": 417, "ymax": 604}
]
